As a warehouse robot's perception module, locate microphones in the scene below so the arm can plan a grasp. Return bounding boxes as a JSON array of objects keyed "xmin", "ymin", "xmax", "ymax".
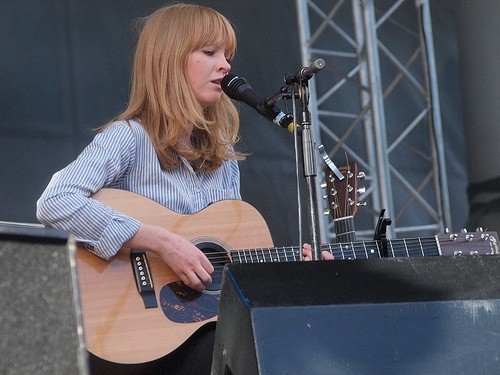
[{"xmin": 221, "ymin": 74, "xmax": 302, "ymax": 134}]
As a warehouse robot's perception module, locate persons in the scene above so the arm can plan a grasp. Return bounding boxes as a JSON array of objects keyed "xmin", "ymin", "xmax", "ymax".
[{"xmin": 36, "ymin": 4, "xmax": 335, "ymax": 375}]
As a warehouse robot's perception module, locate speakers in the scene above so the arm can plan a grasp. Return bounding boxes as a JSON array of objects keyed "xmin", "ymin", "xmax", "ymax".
[{"xmin": 211, "ymin": 254, "xmax": 500, "ymax": 375}]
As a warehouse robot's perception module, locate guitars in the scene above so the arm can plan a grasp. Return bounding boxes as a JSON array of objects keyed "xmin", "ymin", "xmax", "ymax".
[
  {"xmin": 323, "ymin": 163, "xmax": 367, "ymax": 242},
  {"xmin": 70, "ymin": 187, "xmax": 500, "ymax": 365}
]
